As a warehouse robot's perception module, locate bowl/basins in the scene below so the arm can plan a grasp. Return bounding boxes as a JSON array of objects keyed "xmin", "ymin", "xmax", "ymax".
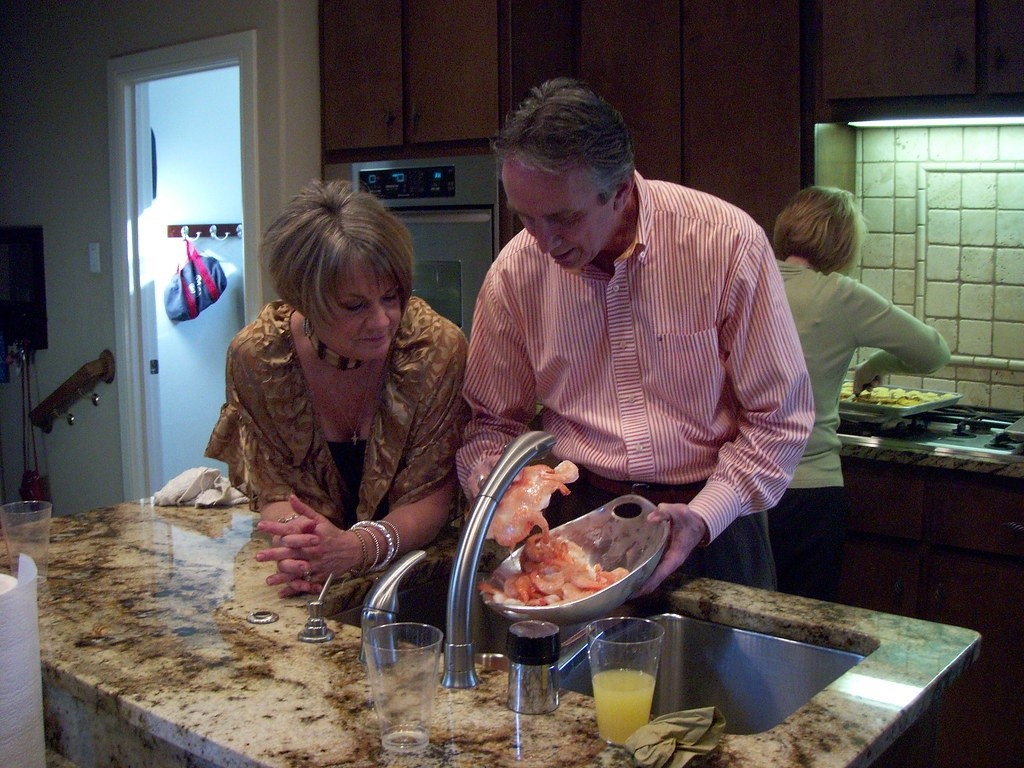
[{"xmin": 483, "ymin": 494, "xmax": 669, "ymax": 626}]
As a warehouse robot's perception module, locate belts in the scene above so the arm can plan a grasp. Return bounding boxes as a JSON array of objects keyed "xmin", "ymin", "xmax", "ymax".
[{"xmin": 574, "ymin": 464, "xmax": 706, "ymax": 505}]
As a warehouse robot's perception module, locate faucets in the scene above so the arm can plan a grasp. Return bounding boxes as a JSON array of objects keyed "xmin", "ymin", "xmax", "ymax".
[{"xmin": 440, "ymin": 430, "xmax": 553, "ymax": 688}]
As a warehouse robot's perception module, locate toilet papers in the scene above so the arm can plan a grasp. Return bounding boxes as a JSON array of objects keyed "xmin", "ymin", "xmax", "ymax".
[{"xmin": 0, "ymin": 552, "xmax": 45, "ymax": 768}]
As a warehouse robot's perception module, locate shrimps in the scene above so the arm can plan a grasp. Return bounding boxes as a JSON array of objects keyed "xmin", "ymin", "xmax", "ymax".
[{"xmin": 478, "ymin": 464, "xmax": 630, "ymax": 607}]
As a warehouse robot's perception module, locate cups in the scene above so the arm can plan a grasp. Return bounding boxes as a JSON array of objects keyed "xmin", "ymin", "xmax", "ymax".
[
  {"xmin": 362, "ymin": 622, "xmax": 444, "ymax": 754},
  {"xmin": 585, "ymin": 616, "xmax": 665, "ymax": 747},
  {"xmin": 0, "ymin": 500, "xmax": 52, "ymax": 584}
]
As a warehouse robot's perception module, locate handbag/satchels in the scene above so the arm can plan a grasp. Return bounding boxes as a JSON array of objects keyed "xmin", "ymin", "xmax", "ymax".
[
  {"xmin": 18, "ymin": 469, "xmax": 46, "ymax": 513},
  {"xmin": 162, "ymin": 236, "xmax": 227, "ymax": 323}
]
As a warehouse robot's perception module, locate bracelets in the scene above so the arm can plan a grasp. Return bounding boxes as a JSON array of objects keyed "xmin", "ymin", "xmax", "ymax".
[
  {"xmin": 277, "ymin": 513, "xmax": 298, "ymax": 523},
  {"xmin": 353, "ymin": 521, "xmax": 395, "ymax": 573},
  {"xmin": 376, "ymin": 520, "xmax": 399, "ymax": 562},
  {"xmin": 356, "ymin": 526, "xmax": 380, "ymax": 571},
  {"xmin": 348, "ymin": 528, "xmax": 367, "ymax": 576}
]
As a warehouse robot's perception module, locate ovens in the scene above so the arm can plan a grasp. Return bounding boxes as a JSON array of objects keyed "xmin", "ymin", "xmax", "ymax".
[{"xmin": 325, "ymin": 153, "xmax": 497, "ymax": 347}]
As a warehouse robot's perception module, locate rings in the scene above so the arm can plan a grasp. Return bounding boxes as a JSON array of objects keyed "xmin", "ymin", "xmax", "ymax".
[
  {"xmin": 475, "ymin": 474, "xmax": 484, "ymax": 485},
  {"xmin": 303, "ymin": 568, "xmax": 313, "ymax": 582}
]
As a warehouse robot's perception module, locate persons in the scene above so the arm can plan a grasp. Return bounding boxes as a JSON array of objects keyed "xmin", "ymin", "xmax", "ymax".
[
  {"xmin": 452, "ymin": 75, "xmax": 817, "ymax": 600},
  {"xmin": 769, "ymin": 185, "xmax": 950, "ymax": 600},
  {"xmin": 200, "ymin": 176, "xmax": 468, "ymax": 599}
]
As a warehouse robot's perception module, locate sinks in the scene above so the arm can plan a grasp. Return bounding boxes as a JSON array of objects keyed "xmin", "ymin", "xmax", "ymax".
[
  {"xmin": 321, "ymin": 535, "xmax": 594, "ymax": 656},
  {"xmin": 545, "ymin": 608, "xmax": 881, "ymax": 746}
]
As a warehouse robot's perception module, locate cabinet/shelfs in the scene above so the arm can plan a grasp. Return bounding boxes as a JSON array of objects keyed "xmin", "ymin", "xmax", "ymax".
[
  {"xmin": 499, "ymin": 0, "xmax": 582, "ymax": 256},
  {"xmin": 318, "ymin": 0, "xmax": 506, "ymax": 164},
  {"xmin": 582, "ymin": 0, "xmax": 857, "ymax": 256},
  {"xmin": 833, "ymin": 457, "xmax": 1024, "ymax": 768},
  {"xmin": 822, "ymin": 0, "xmax": 1024, "ymax": 123}
]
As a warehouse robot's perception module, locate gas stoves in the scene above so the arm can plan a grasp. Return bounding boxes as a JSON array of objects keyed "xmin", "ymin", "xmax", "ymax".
[{"xmin": 838, "ymin": 387, "xmax": 1022, "ymax": 458}]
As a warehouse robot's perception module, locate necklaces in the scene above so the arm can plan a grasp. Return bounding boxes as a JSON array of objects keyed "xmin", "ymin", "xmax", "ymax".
[{"xmin": 319, "ymin": 362, "xmax": 375, "ymax": 446}]
